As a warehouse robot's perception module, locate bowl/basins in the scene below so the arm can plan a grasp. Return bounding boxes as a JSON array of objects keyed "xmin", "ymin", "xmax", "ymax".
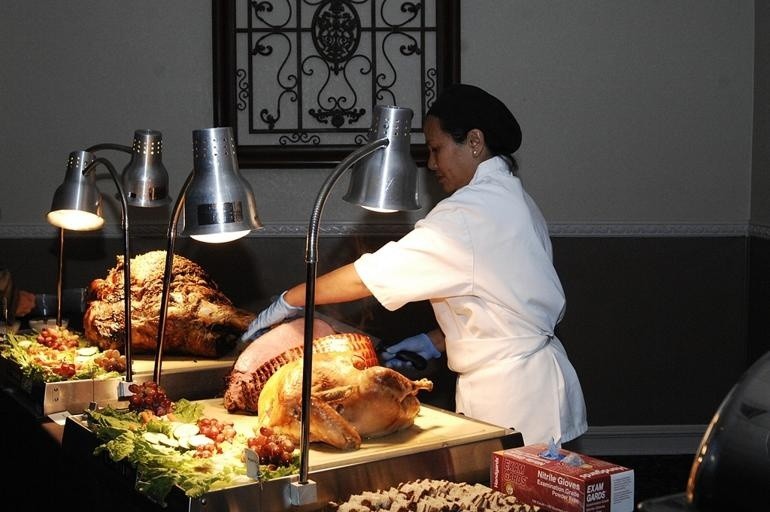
[{"xmin": 28, "ymin": 319, "xmax": 67, "ymax": 334}]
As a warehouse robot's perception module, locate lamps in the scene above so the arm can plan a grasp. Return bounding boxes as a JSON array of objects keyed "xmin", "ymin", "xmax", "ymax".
[
  {"xmin": 42, "ymin": 149, "xmax": 141, "ymax": 397},
  {"xmin": 151, "ymin": 122, "xmax": 263, "ymax": 390},
  {"xmin": 54, "ymin": 126, "xmax": 175, "ymax": 329},
  {"xmin": 292, "ymin": 104, "xmax": 424, "ymax": 506}
]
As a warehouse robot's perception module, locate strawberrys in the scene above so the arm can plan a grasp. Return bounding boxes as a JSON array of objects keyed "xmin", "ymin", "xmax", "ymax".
[{"xmin": 55, "ymin": 362, "xmax": 77, "ymax": 379}]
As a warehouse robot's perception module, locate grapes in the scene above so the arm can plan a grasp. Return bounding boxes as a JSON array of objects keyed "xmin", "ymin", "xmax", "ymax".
[
  {"xmin": 192, "ymin": 418, "xmax": 236, "ymax": 459},
  {"xmin": 94, "ymin": 348, "xmax": 129, "ymax": 373},
  {"xmin": 36, "ymin": 327, "xmax": 66, "ymax": 350},
  {"xmin": 128, "ymin": 380, "xmax": 172, "ymax": 416},
  {"xmin": 242, "ymin": 424, "xmax": 296, "ymax": 467}
]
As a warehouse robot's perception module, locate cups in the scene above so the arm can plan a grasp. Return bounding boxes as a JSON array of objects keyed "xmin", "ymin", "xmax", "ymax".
[
  {"xmin": 63, "ymin": 288, "xmax": 87, "ymax": 323},
  {"xmin": 34, "ymin": 295, "xmax": 58, "ymax": 314}
]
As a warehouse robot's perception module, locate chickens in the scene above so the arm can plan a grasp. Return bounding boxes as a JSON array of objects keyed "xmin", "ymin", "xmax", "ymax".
[{"xmin": 257, "ymin": 351, "xmax": 433, "ymax": 450}]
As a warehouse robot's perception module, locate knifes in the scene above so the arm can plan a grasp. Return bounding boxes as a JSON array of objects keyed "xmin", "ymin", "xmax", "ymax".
[{"xmin": 269, "ymin": 293, "xmax": 428, "ymax": 373}]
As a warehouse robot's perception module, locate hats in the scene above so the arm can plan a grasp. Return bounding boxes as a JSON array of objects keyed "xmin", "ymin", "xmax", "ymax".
[{"xmin": 432, "ymin": 84, "xmax": 522, "ymax": 154}]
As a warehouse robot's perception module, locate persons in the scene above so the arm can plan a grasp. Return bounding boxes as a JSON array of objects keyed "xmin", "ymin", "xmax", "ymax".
[
  {"xmin": 240, "ymin": 79, "xmax": 591, "ymax": 455},
  {"xmin": 1, "ymin": 267, "xmax": 41, "ymax": 331}
]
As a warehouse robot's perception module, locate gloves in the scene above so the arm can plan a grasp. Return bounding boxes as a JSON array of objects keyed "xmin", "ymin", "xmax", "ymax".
[
  {"xmin": 241, "ymin": 288, "xmax": 304, "ymax": 344},
  {"xmin": 381, "ymin": 333, "xmax": 441, "ymax": 370}
]
